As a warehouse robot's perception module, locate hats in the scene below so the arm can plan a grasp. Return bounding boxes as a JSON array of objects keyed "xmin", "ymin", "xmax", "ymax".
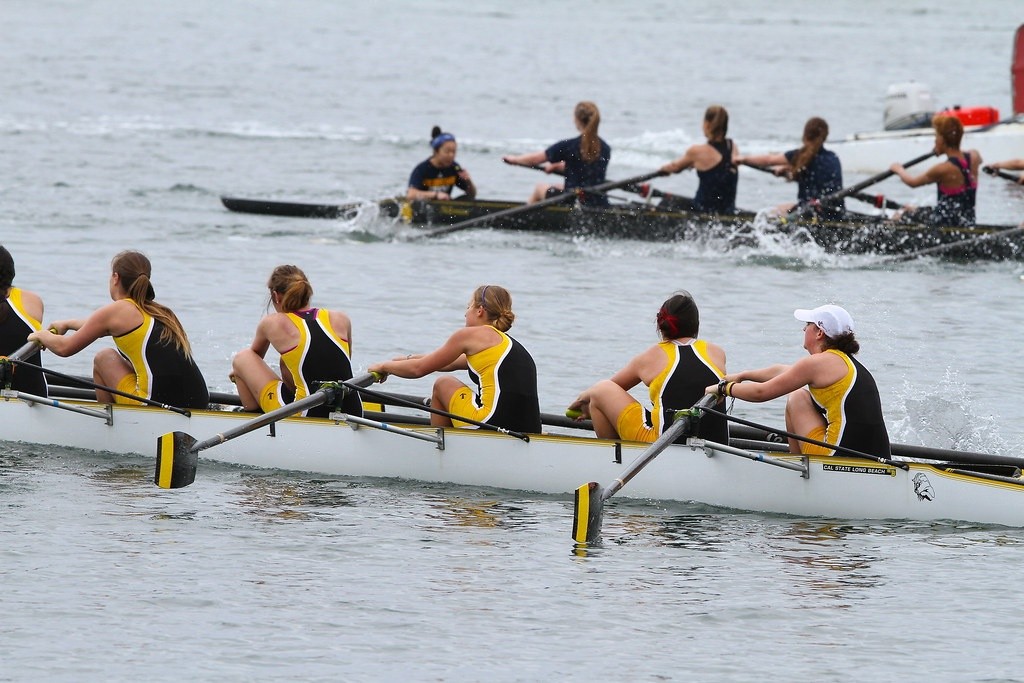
[
  {"xmin": 794, "ymin": 305, "xmax": 855, "ymax": 339},
  {"xmin": 933, "ymin": 114, "xmax": 966, "ymax": 146}
]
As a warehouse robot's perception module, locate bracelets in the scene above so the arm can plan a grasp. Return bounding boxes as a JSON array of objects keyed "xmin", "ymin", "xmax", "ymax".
[
  {"xmin": 434, "ymin": 190, "xmax": 439, "ymax": 199},
  {"xmin": 722, "ymin": 382, "xmax": 736, "ymax": 397},
  {"xmin": 406, "ymin": 355, "xmax": 412, "ymax": 360}
]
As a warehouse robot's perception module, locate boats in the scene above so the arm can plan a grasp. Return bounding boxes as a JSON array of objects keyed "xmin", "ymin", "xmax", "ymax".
[
  {"xmin": 221, "ymin": 191, "xmax": 1024, "ymax": 270},
  {"xmin": 0, "ymin": 374, "xmax": 1023, "ymax": 534}
]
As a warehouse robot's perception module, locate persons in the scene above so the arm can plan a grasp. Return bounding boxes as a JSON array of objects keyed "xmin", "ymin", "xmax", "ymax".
[
  {"xmin": 984, "ymin": 158, "xmax": 1024, "ymax": 185},
  {"xmin": 705, "ymin": 304, "xmax": 892, "ymax": 460},
  {"xmin": 568, "ymin": 290, "xmax": 729, "ymax": 446},
  {"xmin": 228, "ymin": 264, "xmax": 364, "ymax": 418},
  {"xmin": 890, "ymin": 117, "xmax": 981, "ymax": 226},
  {"xmin": 404, "ymin": 125, "xmax": 477, "ymax": 202},
  {"xmin": 0, "ymin": 245, "xmax": 48, "ymax": 400},
  {"xmin": 501, "ymin": 101, "xmax": 612, "ymax": 208},
  {"xmin": 655, "ymin": 105, "xmax": 739, "ymax": 214},
  {"xmin": 27, "ymin": 248, "xmax": 210, "ymax": 409},
  {"xmin": 736, "ymin": 118, "xmax": 845, "ymax": 221},
  {"xmin": 369, "ymin": 284, "xmax": 543, "ymax": 433}
]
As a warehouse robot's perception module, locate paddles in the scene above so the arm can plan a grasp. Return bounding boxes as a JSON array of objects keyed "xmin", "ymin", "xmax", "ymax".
[
  {"xmin": 503, "ymin": 157, "xmax": 669, "ymax": 200},
  {"xmin": 357, "ymin": 385, "xmax": 592, "ymax": 432},
  {"xmin": 726, "ymin": 420, "xmax": 1023, "ymax": 473},
  {"xmin": 568, "ymin": 391, "xmax": 725, "ymax": 543},
  {"xmin": 873, "ymin": 224, "xmax": 1024, "ymax": 264},
  {"xmin": 0, "ymin": 328, "xmax": 57, "ymax": 390},
  {"xmin": 981, "ymin": 163, "xmax": 1022, "ymax": 185},
  {"xmin": 401, "ymin": 169, "xmax": 669, "ymax": 242},
  {"xmin": 728, "ymin": 150, "xmax": 935, "ymax": 250},
  {"xmin": 153, "ymin": 372, "xmax": 391, "ymax": 489},
  {"xmin": 45, "ymin": 370, "xmax": 242, "ymax": 409}
]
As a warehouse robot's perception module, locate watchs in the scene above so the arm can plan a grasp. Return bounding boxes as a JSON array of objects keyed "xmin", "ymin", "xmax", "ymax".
[{"xmin": 717, "ymin": 379, "xmax": 727, "ymax": 395}]
